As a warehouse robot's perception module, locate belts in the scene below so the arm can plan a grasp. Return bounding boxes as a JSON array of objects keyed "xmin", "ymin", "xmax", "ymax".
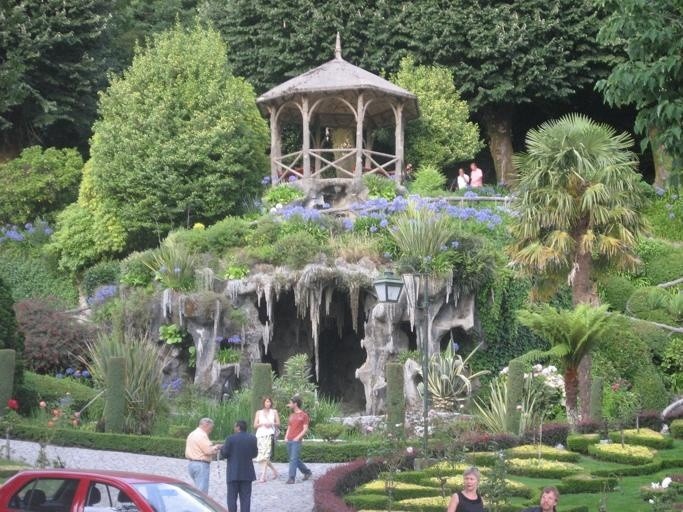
[{"xmin": 191, "ymin": 460, "xmax": 210, "ymax": 464}]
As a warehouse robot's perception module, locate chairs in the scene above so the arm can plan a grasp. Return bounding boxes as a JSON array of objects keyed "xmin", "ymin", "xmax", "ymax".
[{"xmin": 25, "ymin": 490, "xmax": 48, "ymax": 512}]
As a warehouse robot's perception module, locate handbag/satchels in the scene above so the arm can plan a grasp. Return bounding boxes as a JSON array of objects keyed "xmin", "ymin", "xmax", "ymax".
[{"xmin": 275, "ymin": 427, "xmax": 281, "ymax": 437}]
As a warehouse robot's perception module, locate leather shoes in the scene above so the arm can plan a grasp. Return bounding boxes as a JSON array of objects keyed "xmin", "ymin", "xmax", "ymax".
[
  {"xmin": 286, "ymin": 478, "xmax": 295, "ymax": 484},
  {"xmin": 302, "ymin": 471, "xmax": 312, "ymax": 480}
]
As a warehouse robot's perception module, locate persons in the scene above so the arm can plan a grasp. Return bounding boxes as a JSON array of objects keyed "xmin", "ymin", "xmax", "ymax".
[
  {"xmin": 450, "ymin": 168, "xmax": 470, "ymax": 192},
  {"xmin": 518, "ymin": 483, "xmax": 558, "ymax": 512},
  {"xmin": 253, "ymin": 395, "xmax": 280, "ymax": 482},
  {"xmin": 469, "ymin": 162, "xmax": 483, "ymax": 187},
  {"xmin": 447, "ymin": 466, "xmax": 485, "ymax": 511},
  {"xmin": 184, "ymin": 417, "xmax": 221, "ymax": 495},
  {"xmin": 283, "ymin": 396, "xmax": 311, "ymax": 484},
  {"xmin": 219, "ymin": 420, "xmax": 258, "ymax": 512}
]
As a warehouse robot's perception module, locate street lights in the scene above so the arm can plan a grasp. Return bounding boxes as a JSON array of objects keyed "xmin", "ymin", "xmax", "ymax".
[{"xmin": 376, "ymin": 271, "xmax": 433, "ymax": 450}]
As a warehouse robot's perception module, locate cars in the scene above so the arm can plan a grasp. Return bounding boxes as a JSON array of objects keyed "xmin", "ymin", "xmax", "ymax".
[{"xmin": 0, "ymin": 467, "xmax": 229, "ymax": 512}]
